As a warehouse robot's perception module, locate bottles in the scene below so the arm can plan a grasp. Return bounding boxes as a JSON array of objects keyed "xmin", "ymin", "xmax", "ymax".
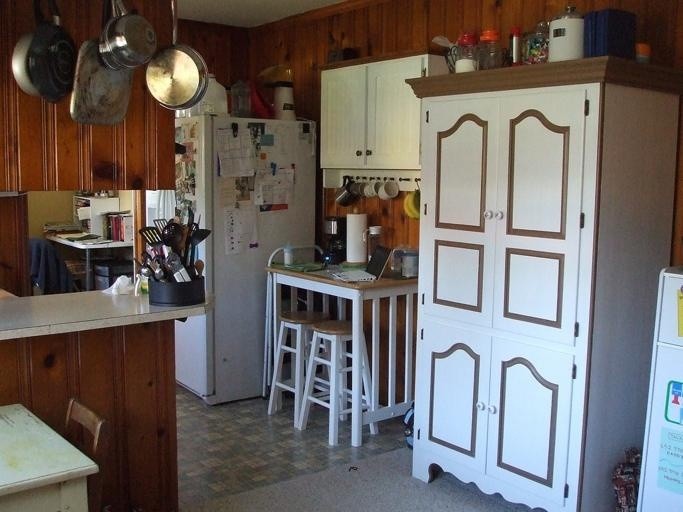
[
  {"xmin": 362, "ymin": 224, "xmax": 386, "ymax": 266},
  {"xmin": 230, "ymin": 79, "xmax": 252, "ymax": 118},
  {"xmin": 453, "ymin": 4, "xmax": 586, "ymax": 74},
  {"xmin": 391, "ymin": 248, "xmax": 419, "ymax": 280}
]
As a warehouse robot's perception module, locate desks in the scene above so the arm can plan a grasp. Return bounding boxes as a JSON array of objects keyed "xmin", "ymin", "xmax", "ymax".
[
  {"xmin": 0, "ymin": 403, "xmax": 101, "ymax": 511},
  {"xmin": 263, "ymin": 260, "xmax": 418, "ymax": 448},
  {"xmin": 46, "ymin": 234, "xmax": 133, "ymax": 290}
]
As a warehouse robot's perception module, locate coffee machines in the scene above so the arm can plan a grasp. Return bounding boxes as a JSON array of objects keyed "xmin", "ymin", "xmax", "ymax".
[{"xmin": 320, "ymin": 216, "xmax": 346, "ymax": 265}]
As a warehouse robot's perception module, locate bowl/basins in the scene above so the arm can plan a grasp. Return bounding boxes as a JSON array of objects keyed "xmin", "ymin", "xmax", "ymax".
[{"xmin": 147, "ymin": 273, "xmax": 205, "ymax": 307}]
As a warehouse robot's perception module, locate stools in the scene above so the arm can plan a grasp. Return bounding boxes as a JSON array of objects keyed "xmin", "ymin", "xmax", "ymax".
[
  {"xmin": 298, "ymin": 320, "xmax": 379, "ymax": 445},
  {"xmin": 267, "ymin": 311, "xmax": 329, "ymax": 429}
]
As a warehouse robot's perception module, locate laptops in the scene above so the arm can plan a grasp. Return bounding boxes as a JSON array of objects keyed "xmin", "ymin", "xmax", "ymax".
[{"xmin": 331, "ymin": 245, "xmax": 393, "ymax": 283}]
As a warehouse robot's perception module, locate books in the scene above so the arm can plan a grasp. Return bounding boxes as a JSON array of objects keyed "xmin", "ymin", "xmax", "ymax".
[{"xmin": 39, "ymin": 190, "xmax": 134, "ymax": 277}]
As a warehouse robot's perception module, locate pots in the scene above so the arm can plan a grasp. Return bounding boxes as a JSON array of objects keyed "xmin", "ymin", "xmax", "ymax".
[{"xmin": 11, "ymin": 0, "xmax": 210, "ymax": 125}]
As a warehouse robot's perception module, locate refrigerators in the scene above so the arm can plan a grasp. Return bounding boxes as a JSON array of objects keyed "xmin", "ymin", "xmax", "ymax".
[{"xmin": 143, "ymin": 112, "xmax": 320, "ymax": 408}]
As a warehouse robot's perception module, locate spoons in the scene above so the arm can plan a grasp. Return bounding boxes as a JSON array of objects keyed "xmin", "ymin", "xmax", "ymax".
[{"xmin": 195, "ymin": 259, "xmax": 205, "ymax": 277}]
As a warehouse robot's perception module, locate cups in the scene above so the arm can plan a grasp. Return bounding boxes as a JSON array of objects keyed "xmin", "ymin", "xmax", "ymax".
[
  {"xmin": 334, "ymin": 176, "xmax": 399, "ymax": 207},
  {"xmin": 273, "ymin": 86, "xmax": 297, "ymax": 121}
]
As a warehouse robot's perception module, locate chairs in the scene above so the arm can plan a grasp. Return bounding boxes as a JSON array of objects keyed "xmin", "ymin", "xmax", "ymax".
[{"xmin": 63, "ymin": 396, "xmax": 106, "ymax": 462}]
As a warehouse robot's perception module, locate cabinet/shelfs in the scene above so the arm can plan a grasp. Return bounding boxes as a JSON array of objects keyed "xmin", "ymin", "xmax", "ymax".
[
  {"xmin": 404, "ymin": 56, "xmax": 683, "ymax": 512},
  {"xmin": 71, "ymin": 194, "xmax": 119, "ymax": 235},
  {"xmin": 321, "ymin": 50, "xmax": 449, "ymax": 170}
]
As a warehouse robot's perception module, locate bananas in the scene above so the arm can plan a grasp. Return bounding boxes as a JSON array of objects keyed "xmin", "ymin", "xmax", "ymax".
[{"xmin": 403, "ymin": 190, "xmax": 419, "ymax": 219}]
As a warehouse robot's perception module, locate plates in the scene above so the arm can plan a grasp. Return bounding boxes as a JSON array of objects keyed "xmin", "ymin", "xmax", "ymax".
[{"xmin": 403, "ymin": 190, "xmax": 420, "ymax": 219}]
